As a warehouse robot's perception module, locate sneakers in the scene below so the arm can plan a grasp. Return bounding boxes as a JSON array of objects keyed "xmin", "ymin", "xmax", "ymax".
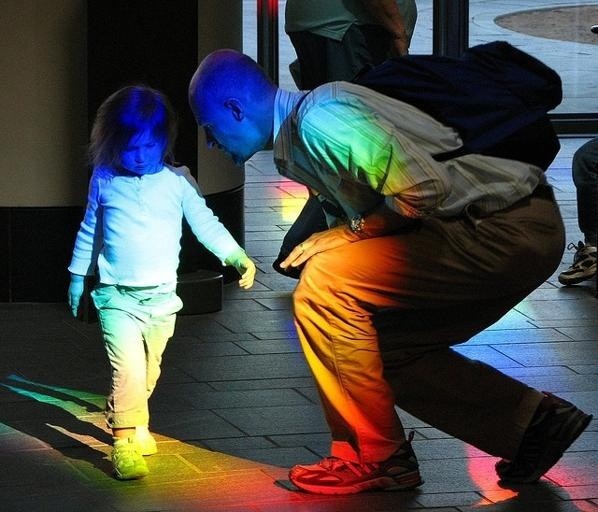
[
  {"xmin": 135, "ymin": 426, "xmax": 158, "ymax": 456},
  {"xmin": 289, "ymin": 430, "xmax": 425, "ymax": 495},
  {"xmin": 111, "ymin": 438, "xmax": 149, "ymax": 480},
  {"xmin": 558, "ymin": 240, "xmax": 598, "ymax": 285},
  {"xmin": 494, "ymin": 390, "xmax": 594, "ymax": 484}
]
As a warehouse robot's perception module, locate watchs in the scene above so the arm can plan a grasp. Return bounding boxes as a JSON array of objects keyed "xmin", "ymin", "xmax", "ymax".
[{"xmin": 348, "ymin": 214, "xmax": 370, "ymax": 241}]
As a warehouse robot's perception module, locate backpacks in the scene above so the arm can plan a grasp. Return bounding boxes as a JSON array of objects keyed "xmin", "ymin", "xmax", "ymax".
[{"xmin": 293, "ymin": 39, "xmax": 563, "ymax": 172}]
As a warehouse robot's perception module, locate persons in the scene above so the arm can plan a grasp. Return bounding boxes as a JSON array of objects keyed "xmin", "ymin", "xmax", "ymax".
[
  {"xmin": 189, "ymin": 49, "xmax": 592, "ymax": 494},
  {"xmin": 559, "ymin": 138, "xmax": 598, "ymax": 283},
  {"xmin": 272, "ymin": 0, "xmax": 417, "ymax": 278},
  {"xmin": 68, "ymin": 86, "xmax": 256, "ymax": 480}
]
